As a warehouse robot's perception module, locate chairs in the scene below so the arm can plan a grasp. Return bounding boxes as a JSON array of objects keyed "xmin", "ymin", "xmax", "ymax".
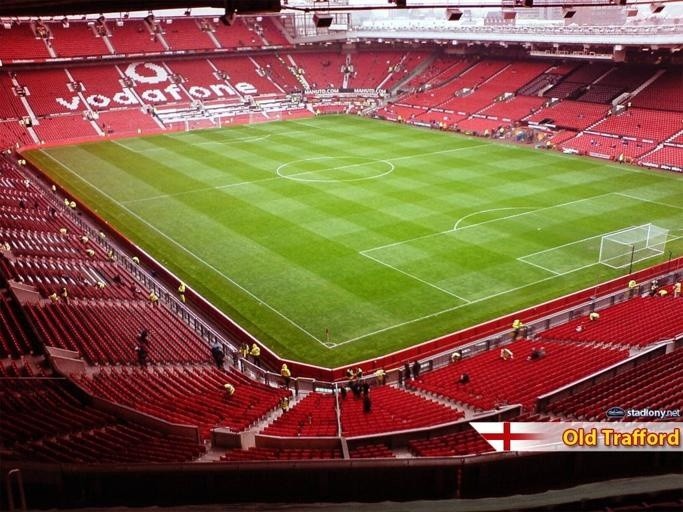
[{"xmin": 1, "ymin": 1, "xmax": 683, "ymax": 511}]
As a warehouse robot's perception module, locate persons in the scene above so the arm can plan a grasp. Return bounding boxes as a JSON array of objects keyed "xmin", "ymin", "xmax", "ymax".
[
  {"xmin": 280, "ymin": 270, "xmax": 683, "ymax": 417},
  {"xmin": 0, "ymin": 159, "xmax": 261, "ymax": 397},
  {"xmin": 1, "ymin": 17, "xmax": 661, "ymax": 167}
]
